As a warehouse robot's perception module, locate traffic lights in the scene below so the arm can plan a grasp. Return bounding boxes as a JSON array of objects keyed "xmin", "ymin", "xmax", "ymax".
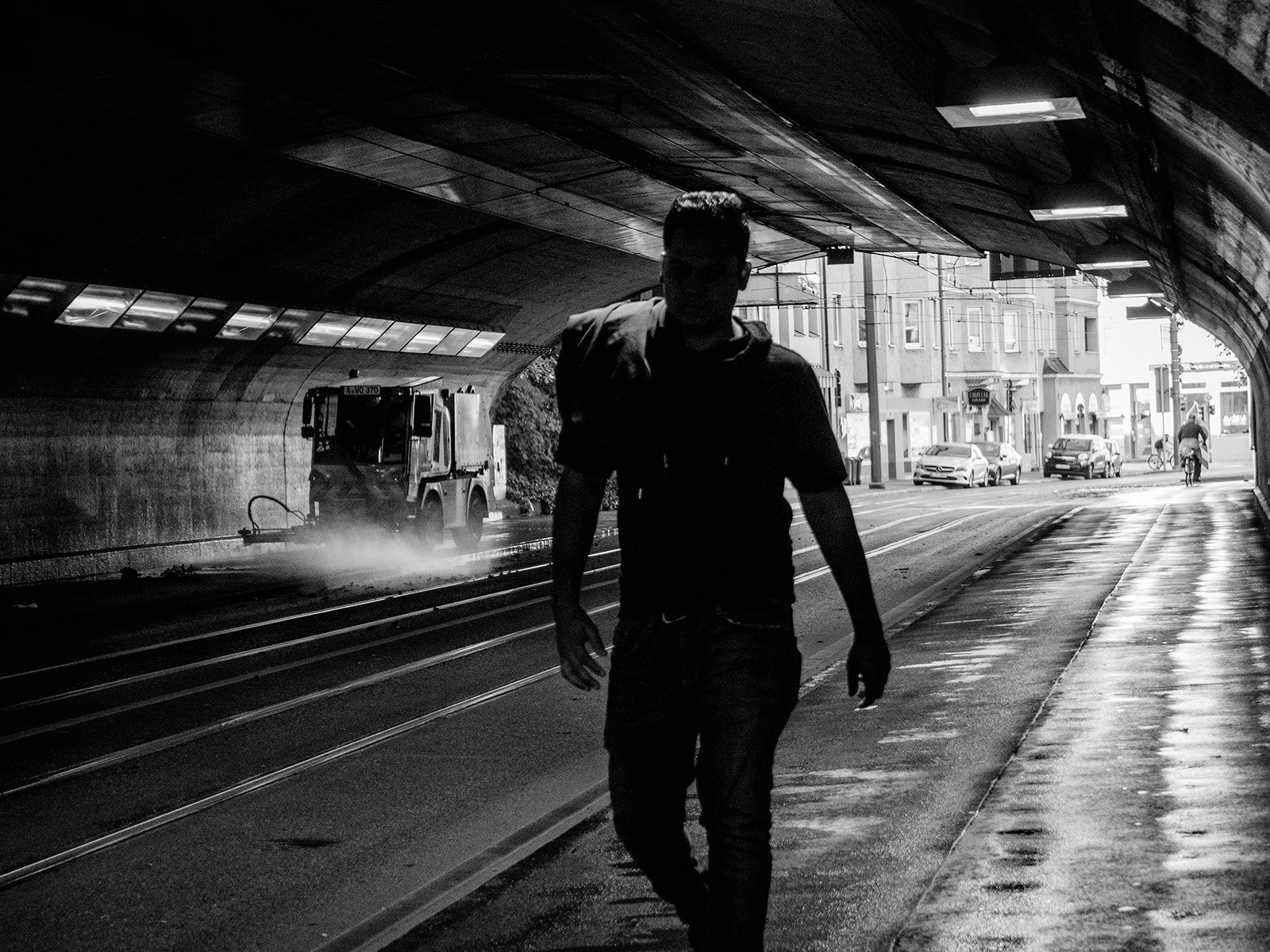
[{"xmin": 1198, "ymin": 407, "xmax": 1204, "ymax": 420}]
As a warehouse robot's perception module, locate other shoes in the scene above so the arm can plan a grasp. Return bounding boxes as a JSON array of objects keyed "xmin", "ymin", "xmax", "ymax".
[{"xmin": 1192, "ymin": 478, "xmax": 1201, "ymax": 483}]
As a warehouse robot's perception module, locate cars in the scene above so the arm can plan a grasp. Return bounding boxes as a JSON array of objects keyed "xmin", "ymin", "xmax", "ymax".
[
  {"xmin": 913, "ymin": 442, "xmax": 989, "ymax": 488},
  {"xmin": 1043, "ymin": 433, "xmax": 1111, "ymax": 479},
  {"xmin": 964, "ymin": 441, "xmax": 1022, "ymax": 486},
  {"xmin": 1104, "ymin": 438, "xmax": 1123, "ymax": 478}
]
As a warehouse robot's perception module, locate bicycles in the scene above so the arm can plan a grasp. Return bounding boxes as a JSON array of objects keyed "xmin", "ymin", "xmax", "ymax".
[
  {"xmin": 1181, "ymin": 441, "xmax": 1205, "ymax": 487},
  {"xmin": 1147, "ymin": 444, "xmax": 1175, "ymax": 470}
]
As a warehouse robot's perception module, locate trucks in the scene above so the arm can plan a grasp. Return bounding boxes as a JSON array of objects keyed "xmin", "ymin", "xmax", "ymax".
[{"xmin": 235, "ymin": 368, "xmax": 498, "ymax": 554}]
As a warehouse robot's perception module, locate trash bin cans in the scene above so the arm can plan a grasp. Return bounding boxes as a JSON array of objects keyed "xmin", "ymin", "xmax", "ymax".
[{"xmin": 842, "ymin": 457, "xmax": 863, "ymax": 485}]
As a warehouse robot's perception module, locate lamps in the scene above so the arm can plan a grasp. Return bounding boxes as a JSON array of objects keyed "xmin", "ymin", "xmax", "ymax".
[{"xmin": 932, "ymin": 60, "xmax": 1172, "ymax": 320}]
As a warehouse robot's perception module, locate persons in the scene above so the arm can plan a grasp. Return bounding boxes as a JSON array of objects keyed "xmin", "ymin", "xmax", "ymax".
[
  {"xmin": 1177, "ymin": 414, "xmax": 1207, "ymax": 483},
  {"xmin": 547, "ymin": 188, "xmax": 892, "ymax": 952},
  {"xmin": 1154, "ymin": 434, "xmax": 1173, "ymax": 471}
]
